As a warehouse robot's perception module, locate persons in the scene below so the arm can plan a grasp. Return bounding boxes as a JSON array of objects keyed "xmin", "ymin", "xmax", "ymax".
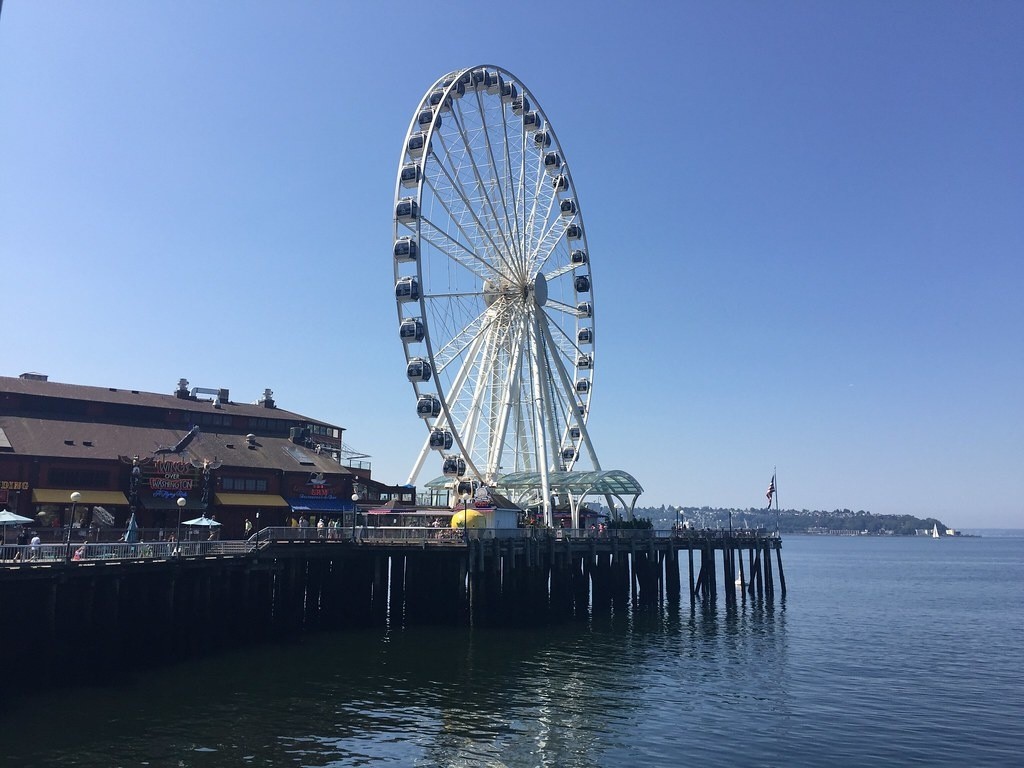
[
  {"xmin": 28, "ymin": 533, "xmax": 41, "ymax": 562},
  {"xmin": 13, "ymin": 529, "xmax": 29, "ymax": 562},
  {"xmin": 75, "ymin": 541, "xmax": 91, "ymax": 558},
  {"xmin": 207, "ymin": 515, "xmax": 695, "ymax": 540}
]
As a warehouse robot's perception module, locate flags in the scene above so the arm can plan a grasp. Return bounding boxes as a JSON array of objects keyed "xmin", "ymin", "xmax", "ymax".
[{"xmin": 766, "ymin": 475, "xmax": 775, "ymax": 509}]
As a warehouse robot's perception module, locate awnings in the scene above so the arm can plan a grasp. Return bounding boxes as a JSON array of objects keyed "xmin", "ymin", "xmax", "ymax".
[{"xmin": 31, "ymin": 489, "xmax": 350, "ymax": 512}]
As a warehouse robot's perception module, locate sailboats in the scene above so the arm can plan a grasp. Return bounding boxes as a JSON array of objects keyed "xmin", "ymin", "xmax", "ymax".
[{"xmin": 932, "ymin": 523, "xmax": 941, "ymax": 539}]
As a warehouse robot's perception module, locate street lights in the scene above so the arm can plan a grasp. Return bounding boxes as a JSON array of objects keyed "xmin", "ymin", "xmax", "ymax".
[
  {"xmin": 351, "ymin": 492, "xmax": 360, "ymax": 544},
  {"xmin": 63, "ymin": 490, "xmax": 81, "ymax": 558},
  {"xmin": 679, "ymin": 509, "xmax": 685, "ymax": 530},
  {"xmin": 613, "ymin": 503, "xmax": 619, "ymax": 536},
  {"xmin": 176, "ymin": 497, "xmax": 187, "ymax": 557},
  {"xmin": 255, "ymin": 512, "xmax": 261, "ymax": 549},
  {"xmin": 462, "ymin": 492, "xmax": 469, "ymax": 536}
]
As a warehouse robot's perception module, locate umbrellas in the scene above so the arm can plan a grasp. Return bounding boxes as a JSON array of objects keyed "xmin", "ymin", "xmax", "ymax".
[
  {"xmin": 181, "ymin": 515, "xmax": 222, "ymax": 526},
  {"xmin": 0, "ymin": 509, "xmax": 34, "ymax": 544}
]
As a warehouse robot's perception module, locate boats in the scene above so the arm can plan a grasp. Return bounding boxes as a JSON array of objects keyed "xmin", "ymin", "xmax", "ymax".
[{"xmin": 735, "ymin": 570, "xmax": 750, "ymax": 586}]
